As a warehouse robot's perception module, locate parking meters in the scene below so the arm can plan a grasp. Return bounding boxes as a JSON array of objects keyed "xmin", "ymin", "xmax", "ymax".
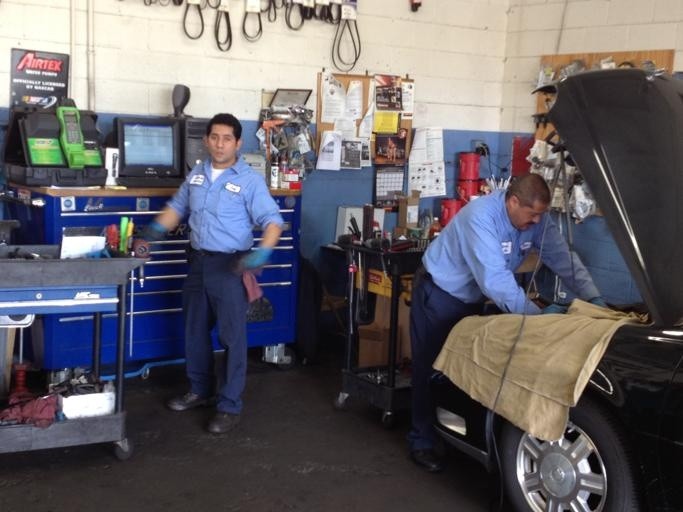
[{"xmin": 57, "ymin": 97, "xmax": 86, "ymax": 170}]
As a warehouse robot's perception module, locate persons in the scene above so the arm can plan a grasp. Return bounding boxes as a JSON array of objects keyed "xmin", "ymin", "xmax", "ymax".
[
  {"xmin": 410, "ymin": 172, "xmax": 610, "ymax": 473},
  {"xmin": 136, "ymin": 113, "xmax": 285, "ymax": 433}
]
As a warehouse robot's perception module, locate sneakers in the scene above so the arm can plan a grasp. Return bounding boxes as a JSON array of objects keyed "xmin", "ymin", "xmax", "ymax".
[
  {"xmin": 207, "ymin": 411, "xmax": 241, "ymax": 434},
  {"xmin": 166, "ymin": 391, "xmax": 217, "ymax": 411}
]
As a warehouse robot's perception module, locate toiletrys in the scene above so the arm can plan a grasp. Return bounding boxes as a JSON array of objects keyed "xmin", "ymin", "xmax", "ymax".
[{"xmin": 104, "ymin": 146, "xmax": 121, "ymax": 187}]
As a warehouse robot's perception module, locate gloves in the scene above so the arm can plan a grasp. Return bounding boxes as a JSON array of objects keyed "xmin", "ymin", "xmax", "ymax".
[
  {"xmin": 541, "ymin": 302, "xmax": 567, "ymax": 315},
  {"xmin": 244, "ymin": 246, "xmax": 273, "ymax": 270},
  {"xmin": 591, "ymin": 297, "xmax": 608, "ymax": 307}
]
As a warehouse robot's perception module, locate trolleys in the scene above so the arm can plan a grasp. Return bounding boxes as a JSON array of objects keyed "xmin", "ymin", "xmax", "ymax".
[
  {"xmin": 0, "ymin": 242, "xmax": 150, "ymax": 461},
  {"xmin": 334, "ymin": 238, "xmax": 427, "ymax": 432}
]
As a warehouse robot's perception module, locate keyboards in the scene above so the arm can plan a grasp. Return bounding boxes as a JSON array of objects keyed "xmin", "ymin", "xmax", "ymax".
[{"xmin": 115, "ymin": 178, "xmax": 185, "ymax": 187}]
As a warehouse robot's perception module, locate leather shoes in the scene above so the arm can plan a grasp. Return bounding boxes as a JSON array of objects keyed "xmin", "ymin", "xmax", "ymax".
[{"xmin": 409, "ymin": 447, "xmax": 444, "ymax": 473}]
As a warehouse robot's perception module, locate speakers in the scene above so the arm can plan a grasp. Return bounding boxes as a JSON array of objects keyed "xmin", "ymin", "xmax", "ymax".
[{"xmin": 168, "ymin": 85, "xmax": 193, "ymax": 118}]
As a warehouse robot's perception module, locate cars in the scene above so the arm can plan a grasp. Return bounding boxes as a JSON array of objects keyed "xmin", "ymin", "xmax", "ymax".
[{"xmin": 426, "ymin": 67, "xmax": 682, "ymax": 508}]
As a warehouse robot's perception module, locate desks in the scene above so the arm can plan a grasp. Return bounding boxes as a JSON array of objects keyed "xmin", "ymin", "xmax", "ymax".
[{"xmin": 5, "ymin": 183, "xmax": 302, "ymax": 380}]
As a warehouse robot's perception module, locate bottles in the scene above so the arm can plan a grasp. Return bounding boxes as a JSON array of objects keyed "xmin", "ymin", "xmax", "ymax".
[
  {"xmin": 430, "ymin": 217, "xmax": 441, "ymax": 241},
  {"xmin": 373, "ymin": 222, "xmax": 381, "ymax": 238},
  {"xmin": 271, "ymin": 150, "xmax": 288, "ymax": 188}
]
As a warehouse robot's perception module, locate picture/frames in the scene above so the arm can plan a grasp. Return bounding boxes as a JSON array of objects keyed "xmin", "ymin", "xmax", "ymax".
[{"xmin": 268, "ymin": 89, "xmax": 313, "ymax": 107}]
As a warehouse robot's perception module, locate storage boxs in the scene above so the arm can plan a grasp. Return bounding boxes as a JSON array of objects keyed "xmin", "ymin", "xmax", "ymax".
[{"xmin": 48, "ymin": 383, "xmax": 116, "ymax": 419}]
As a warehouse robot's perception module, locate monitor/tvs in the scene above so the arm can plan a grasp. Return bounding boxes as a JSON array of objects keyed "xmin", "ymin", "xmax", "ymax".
[{"xmin": 105, "ymin": 118, "xmax": 181, "ymax": 177}]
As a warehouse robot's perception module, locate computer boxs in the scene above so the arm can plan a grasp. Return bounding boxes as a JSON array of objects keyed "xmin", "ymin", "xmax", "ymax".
[{"xmin": 181, "ymin": 118, "xmax": 211, "ymax": 180}]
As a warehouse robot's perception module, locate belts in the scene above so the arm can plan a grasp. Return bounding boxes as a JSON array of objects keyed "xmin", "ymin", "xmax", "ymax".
[
  {"xmin": 187, "ymin": 247, "xmax": 247, "ymax": 258},
  {"xmin": 418, "ymin": 263, "xmax": 434, "ymax": 286}
]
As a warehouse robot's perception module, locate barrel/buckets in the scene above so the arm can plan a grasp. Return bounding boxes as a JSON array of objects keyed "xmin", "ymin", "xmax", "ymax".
[{"xmin": 441, "ymin": 152, "xmax": 488, "ymax": 228}]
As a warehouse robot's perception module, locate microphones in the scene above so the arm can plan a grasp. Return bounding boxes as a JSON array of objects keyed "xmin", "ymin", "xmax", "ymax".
[{"xmin": 113, "ymin": 153, "xmax": 117, "ymax": 177}]
